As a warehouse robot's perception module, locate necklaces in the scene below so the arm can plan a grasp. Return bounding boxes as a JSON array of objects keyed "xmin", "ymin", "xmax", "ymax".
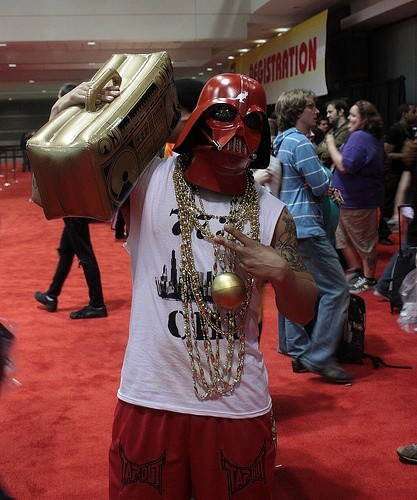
[{"xmin": 173, "ymin": 151, "xmax": 261, "ymax": 401}]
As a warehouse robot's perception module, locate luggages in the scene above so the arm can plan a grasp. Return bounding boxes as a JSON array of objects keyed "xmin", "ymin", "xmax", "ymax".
[{"xmin": 390, "ymin": 204, "xmax": 417, "ymax": 314}]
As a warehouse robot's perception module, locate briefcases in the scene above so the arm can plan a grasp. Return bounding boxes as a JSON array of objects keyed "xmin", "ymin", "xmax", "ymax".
[{"xmin": 26, "ymin": 51, "xmax": 181, "ymax": 222}]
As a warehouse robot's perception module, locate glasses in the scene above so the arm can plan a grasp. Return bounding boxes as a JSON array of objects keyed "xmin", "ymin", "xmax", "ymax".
[{"xmin": 206, "ymin": 104, "xmax": 262, "ymax": 130}]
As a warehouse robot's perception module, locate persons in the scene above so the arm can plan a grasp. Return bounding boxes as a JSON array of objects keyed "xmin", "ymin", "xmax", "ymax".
[
  {"xmin": 30, "ymin": 73, "xmax": 318, "ymax": 500},
  {"xmin": 271, "ymin": 88, "xmax": 355, "ymax": 383},
  {"xmin": 20, "ymin": 133, "xmax": 30, "ymax": 173},
  {"xmin": 305, "ymin": 99, "xmax": 417, "ymax": 465},
  {"xmin": 35, "ymin": 78, "xmax": 206, "ymax": 319}
]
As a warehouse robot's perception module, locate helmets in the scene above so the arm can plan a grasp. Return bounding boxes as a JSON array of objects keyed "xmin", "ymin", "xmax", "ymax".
[{"xmin": 172, "ymin": 73, "xmax": 271, "ymax": 169}]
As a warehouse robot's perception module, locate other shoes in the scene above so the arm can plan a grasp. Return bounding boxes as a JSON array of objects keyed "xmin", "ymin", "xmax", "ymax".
[
  {"xmin": 379, "ymin": 237, "xmax": 392, "ymax": 245},
  {"xmin": 396, "ymin": 444, "xmax": 417, "ymax": 462},
  {"xmin": 345, "ymin": 270, "xmax": 363, "ymax": 283},
  {"xmin": 291, "ymin": 359, "xmax": 351, "ymax": 382},
  {"xmin": 347, "ymin": 277, "xmax": 377, "ymax": 293},
  {"xmin": 70, "ymin": 305, "xmax": 107, "ymax": 319},
  {"xmin": 374, "ymin": 291, "xmax": 390, "ymax": 302},
  {"xmin": 34, "ymin": 291, "xmax": 57, "ymax": 311}
]
